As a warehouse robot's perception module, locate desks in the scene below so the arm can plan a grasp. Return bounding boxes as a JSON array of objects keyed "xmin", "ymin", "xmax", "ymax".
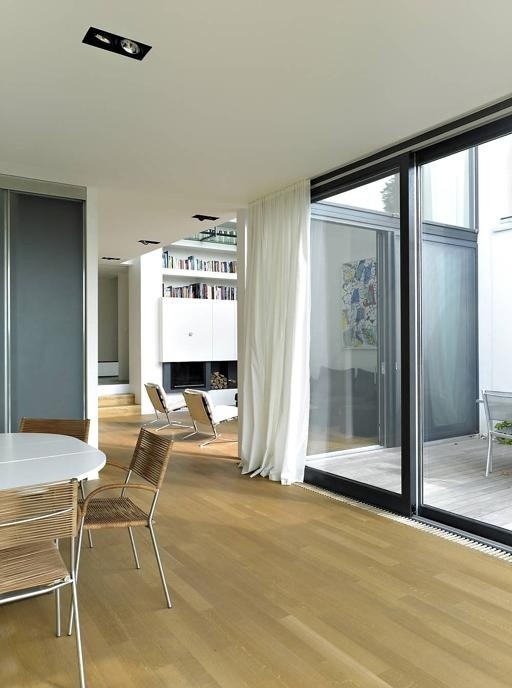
[{"xmin": 0, "ymin": 432, "xmax": 106, "ymax": 489}]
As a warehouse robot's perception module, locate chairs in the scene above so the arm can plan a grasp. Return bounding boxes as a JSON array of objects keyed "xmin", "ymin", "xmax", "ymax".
[
  {"xmin": 67, "ymin": 429, "xmax": 174, "ymax": 636},
  {"xmin": 140, "ymin": 382, "xmax": 193, "ymax": 437},
  {"xmin": 476, "ymin": 390, "xmax": 512, "ymax": 478},
  {"xmin": 183, "ymin": 388, "xmax": 238, "ymax": 447},
  {"xmin": 18, "ymin": 418, "xmax": 94, "ymax": 547},
  {"xmin": 0, "ymin": 477, "xmax": 87, "ymax": 688}
]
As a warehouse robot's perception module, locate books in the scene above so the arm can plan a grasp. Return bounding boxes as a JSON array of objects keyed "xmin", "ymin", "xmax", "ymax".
[
  {"xmin": 163, "ymin": 250, "xmax": 237, "ymax": 273},
  {"xmin": 162, "ymin": 283, "xmax": 234, "ymax": 300}
]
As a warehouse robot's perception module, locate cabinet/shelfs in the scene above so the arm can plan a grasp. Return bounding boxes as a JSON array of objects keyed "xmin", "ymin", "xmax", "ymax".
[
  {"xmin": 162, "ymin": 247, "xmax": 238, "ymax": 300},
  {"xmin": 161, "ymin": 298, "xmax": 238, "ymax": 363}
]
